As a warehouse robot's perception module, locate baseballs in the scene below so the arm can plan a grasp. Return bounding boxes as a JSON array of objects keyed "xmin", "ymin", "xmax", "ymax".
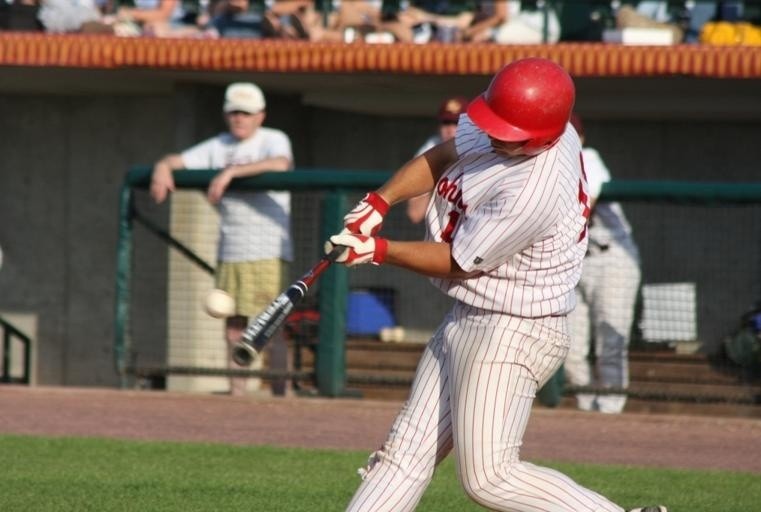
[{"xmin": 203, "ymin": 287, "xmax": 235, "ymax": 318}]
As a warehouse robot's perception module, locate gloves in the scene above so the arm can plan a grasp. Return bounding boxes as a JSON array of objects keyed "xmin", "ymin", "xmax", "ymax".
[
  {"xmin": 344, "ymin": 191, "xmax": 391, "ymax": 236},
  {"xmin": 324, "ymin": 234, "xmax": 387, "ymax": 266}
]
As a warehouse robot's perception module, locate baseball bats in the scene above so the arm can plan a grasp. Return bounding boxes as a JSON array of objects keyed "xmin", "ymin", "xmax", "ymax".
[{"xmin": 233, "ymin": 245, "xmax": 347, "ymax": 366}]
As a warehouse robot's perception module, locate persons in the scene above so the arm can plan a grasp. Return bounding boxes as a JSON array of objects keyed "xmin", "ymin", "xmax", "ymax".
[
  {"xmin": 406, "ymin": 96, "xmax": 469, "ymax": 224},
  {"xmin": 564, "ymin": 114, "xmax": 642, "ymax": 414},
  {"xmin": 149, "ymin": 81, "xmax": 294, "ymax": 399},
  {"xmin": 324, "ymin": 56, "xmax": 668, "ymax": 512},
  {"xmin": 0, "ymin": 0, "xmax": 761, "ymax": 46}
]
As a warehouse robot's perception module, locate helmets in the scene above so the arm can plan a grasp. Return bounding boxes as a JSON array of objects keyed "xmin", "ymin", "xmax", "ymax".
[{"xmin": 467, "ymin": 58, "xmax": 575, "ymax": 157}]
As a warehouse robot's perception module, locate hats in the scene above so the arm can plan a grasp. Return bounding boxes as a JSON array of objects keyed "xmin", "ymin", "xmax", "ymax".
[{"xmin": 222, "ymin": 82, "xmax": 267, "ymax": 115}]
